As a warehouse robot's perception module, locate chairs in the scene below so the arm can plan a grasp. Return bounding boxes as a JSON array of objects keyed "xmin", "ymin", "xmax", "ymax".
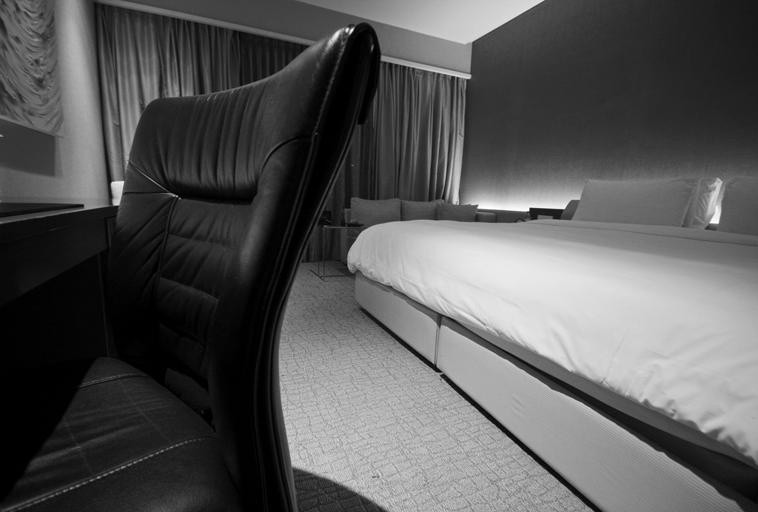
[{"xmin": 0, "ymin": 22, "xmax": 382, "ymax": 512}]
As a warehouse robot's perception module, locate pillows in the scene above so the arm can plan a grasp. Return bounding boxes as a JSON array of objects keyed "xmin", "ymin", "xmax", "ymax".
[
  {"xmin": 571, "ymin": 176, "xmax": 758, "ymax": 236},
  {"xmin": 350, "ymin": 197, "xmax": 478, "ymax": 226}
]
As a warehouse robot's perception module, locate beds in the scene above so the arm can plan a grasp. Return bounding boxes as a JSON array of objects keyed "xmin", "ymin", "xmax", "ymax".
[{"xmin": 353, "ymin": 198, "xmax": 757, "ymax": 512}]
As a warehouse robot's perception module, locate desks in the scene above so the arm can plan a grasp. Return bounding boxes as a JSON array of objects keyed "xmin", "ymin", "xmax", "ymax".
[
  {"xmin": 307, "ymin": 224, "xmax": 363, "ymax": 281},
  {"xmin": 1, "ymin": 204, "xmax": 119, "ymax": 358}
]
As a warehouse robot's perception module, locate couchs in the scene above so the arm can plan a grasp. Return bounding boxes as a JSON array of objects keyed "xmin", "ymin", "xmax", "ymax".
[{"xmin": 337, "ymin": 208, "xmax": 496, "ymax": 271}]
